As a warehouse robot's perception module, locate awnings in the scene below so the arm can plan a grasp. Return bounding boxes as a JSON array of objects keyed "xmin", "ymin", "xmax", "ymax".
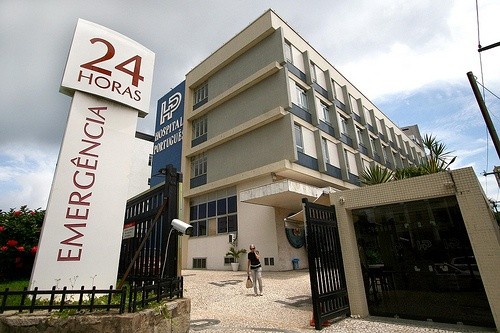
[{"xmin": 283, "ymin": 192, "xmax": 330, "ymax": 229}]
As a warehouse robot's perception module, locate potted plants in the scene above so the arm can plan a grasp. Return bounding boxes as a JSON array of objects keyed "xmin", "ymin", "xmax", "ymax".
[{"xmin": 225, "ymin": 247, "xmax": 247, "ymax": 272}]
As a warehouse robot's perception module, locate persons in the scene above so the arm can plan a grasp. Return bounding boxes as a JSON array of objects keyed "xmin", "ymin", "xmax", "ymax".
[{"xmin": 247, "ymin": 244, "xmax": 263, "ymax": 296}]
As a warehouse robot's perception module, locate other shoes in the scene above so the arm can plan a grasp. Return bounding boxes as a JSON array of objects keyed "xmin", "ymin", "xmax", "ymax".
[
  {"xmin": 255, "ymin": 293, "xmax": 258, "ymax": 296},
  {"xmin": 260, "ymin": 293, "xmax": 263, "ymax": 296}
]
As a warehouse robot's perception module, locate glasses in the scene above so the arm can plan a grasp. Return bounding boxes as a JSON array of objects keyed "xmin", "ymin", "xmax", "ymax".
[{"xmin": 250, "ymin": 248, "xmax": 254, "ymax": 250}]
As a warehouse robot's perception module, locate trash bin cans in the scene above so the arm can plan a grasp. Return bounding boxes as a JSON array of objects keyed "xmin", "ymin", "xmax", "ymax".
[{"xmin": 292, "ymin": 258, "xmax": 301, "ymax": 270}]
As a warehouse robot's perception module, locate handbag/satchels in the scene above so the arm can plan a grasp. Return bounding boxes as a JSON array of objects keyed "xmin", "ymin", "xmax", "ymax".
[{"xmin": 246, "ymin": 276, "xmax": 253, "ymax": 288}]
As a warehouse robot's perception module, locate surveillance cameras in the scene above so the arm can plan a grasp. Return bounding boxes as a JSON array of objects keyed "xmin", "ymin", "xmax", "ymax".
[{"xmin": 171, "ymin": 219, "xmax": 194, "ymax": 235}]
